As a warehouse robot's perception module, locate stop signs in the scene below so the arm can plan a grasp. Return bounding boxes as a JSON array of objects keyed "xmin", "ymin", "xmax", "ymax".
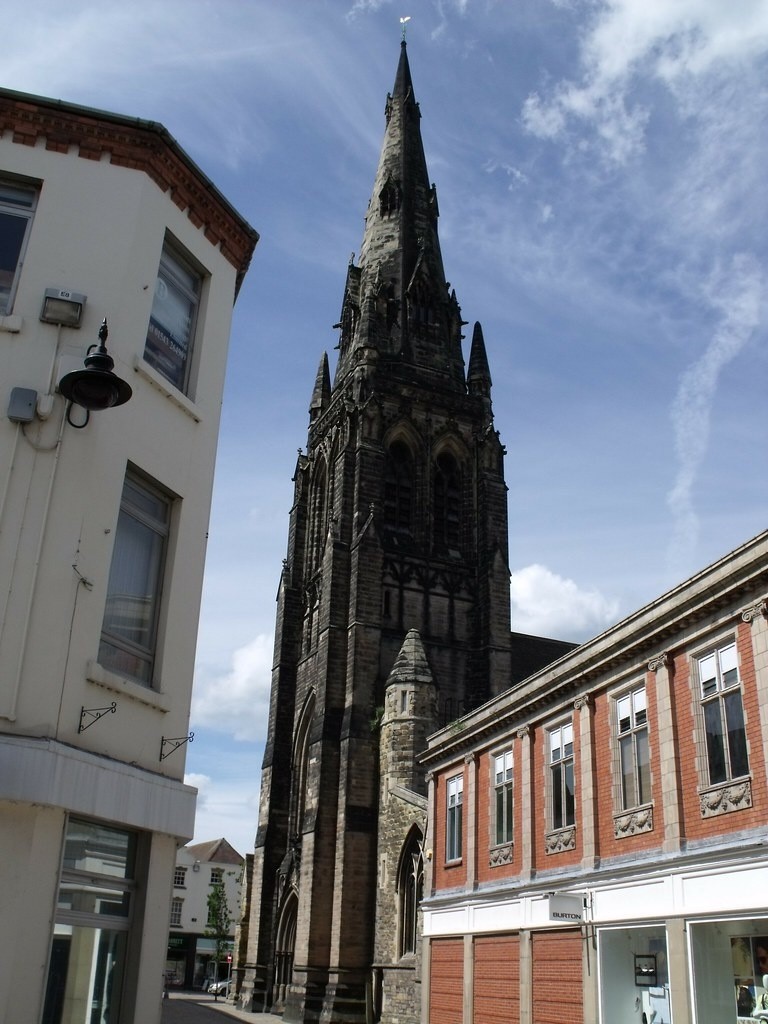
[{"xmin": 227, "ymin": 954, "xmax": 232, "ymax": 964}]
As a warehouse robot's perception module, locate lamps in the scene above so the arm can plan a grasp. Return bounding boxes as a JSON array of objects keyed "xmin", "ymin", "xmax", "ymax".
[{"xmin": 60, "ymin": 317, "xmax": 133, "ymax": 429}]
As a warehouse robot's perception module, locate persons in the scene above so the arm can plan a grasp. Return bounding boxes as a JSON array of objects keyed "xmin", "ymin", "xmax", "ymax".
[{"xmin": 754, "ymin": 974, "xmax": 768, "ymax": 1024}]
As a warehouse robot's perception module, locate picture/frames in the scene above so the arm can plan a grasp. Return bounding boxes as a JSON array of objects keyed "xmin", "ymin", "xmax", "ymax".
[{"xmin": 634, "ymin": 955, "xmax": 657, "ymax": 987}]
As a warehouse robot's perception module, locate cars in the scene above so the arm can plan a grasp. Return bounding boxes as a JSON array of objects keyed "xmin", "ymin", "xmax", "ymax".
[{"xmin": 208, "ymin": 977, "xmax": 233, "ymax": 997}]
